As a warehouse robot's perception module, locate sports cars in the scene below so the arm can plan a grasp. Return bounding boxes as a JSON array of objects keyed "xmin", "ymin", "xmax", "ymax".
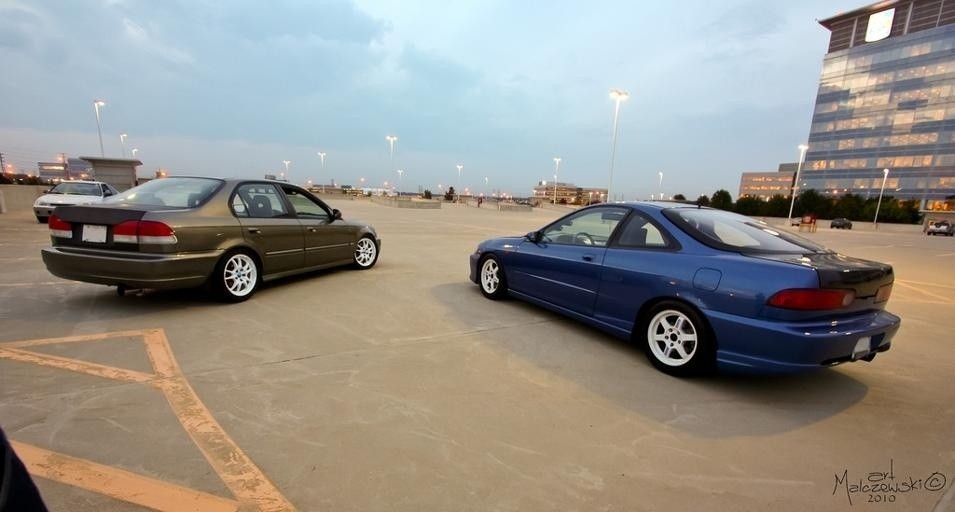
[{"xmin": 466, "ymin": 199, "xmax": 903, "ymax": 383}]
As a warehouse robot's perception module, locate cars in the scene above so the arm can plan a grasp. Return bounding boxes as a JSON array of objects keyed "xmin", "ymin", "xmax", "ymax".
[
  {"xmin": 791, "ymin": 216, "xmax": 803, "ymax": 226},
  {"xmin": 32, "ymin": 181, "xmax": 119, "ymax": 222},
  {"xmin": 928, "ymin": 219, "xmax": 954, "ymax": 236},
  {"xmin": 40, "ymin": 174, "xmax": 381, "ymax": 302},
  {"xmin": 831, "ymin": 217, "xmax": 852, "ymax": 230}
]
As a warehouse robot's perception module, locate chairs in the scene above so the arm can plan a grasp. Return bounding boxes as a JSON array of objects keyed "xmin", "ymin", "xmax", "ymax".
[
  {"xmin": 620, "ymin": 229, "xmax": 646, "ymax": 246},
  {"xmin": 250, "ymin": 195, "xmax": 273, "ymax": 216}
]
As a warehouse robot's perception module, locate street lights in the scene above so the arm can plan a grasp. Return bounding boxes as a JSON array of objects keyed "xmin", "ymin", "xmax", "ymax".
[
  {"xmin": 282, "ymin": 160, "xmax": 291, "ymax": 182},
  {"xmin": 784, "ymin": 143, "xmax": 810, "ymax": 220},
  {"xmin": 316, "ymin": 152, "xmax": 326, "ymax": 193},
  {"xmin": 651, "ymin": 171, "xmax": 672, "ymax": 200},
  {"xmin": 397, "ymin": 169, "xmax": 404, "ymax": 197},
  {"xmin": 552, "ymin": 158, "xmax": 563, "ymax": 206},
  {"xmin": 92, "ymin": 100, "xmax": 139, "ymax": 159},
  {"xmin": 874, "ymin": 167, "xmax": 890, "ymax": 227},
  {"xmin": 485, "ymin": 176, "xmax": 488, "ymax": 200},
  {"xmin": 384, "ymin": 136, "xmax": 398, "ymax": 159},
  {"xmin": 606, "ymin": 90, "xmax": 628, "ymax": 204},
  {"xmin": 455, "ymin": 165, "xmax": 463, "ymax": 200}
]
